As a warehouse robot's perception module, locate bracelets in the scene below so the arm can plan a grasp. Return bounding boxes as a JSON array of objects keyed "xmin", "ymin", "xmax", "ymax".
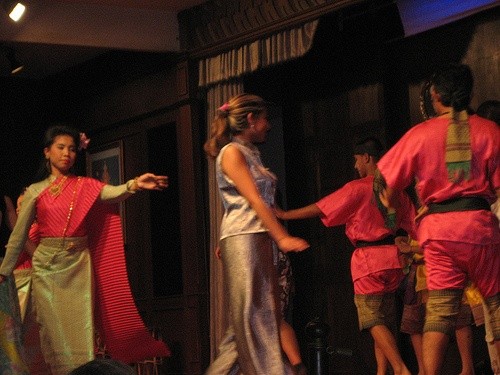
[
  {"xmin": 275, "ymin": 235, "xmax": 291, "ymax": 243},
  {"xmin": 135, "ymin": 178, "xmax": 144, "ymax": 191}
]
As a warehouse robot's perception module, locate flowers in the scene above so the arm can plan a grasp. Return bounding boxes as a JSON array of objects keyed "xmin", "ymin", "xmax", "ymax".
[{"xmin": 80, "ymin": 132, "xmax": 89, "ymax": 150}]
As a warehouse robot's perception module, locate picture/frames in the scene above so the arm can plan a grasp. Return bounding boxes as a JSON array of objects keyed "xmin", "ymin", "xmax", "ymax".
[{"xmin": 84, "ymin": 140, "xmax": 127, "ymax": 245}]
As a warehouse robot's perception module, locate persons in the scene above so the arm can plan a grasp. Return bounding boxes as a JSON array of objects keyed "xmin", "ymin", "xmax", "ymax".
[
  {"xmin": 0, "ymin": 65, "xmax": 500, "ymax": 375},
  {"xmin": 204, "ymin": 93, "xmax": 310, "ymax": 375}
]
